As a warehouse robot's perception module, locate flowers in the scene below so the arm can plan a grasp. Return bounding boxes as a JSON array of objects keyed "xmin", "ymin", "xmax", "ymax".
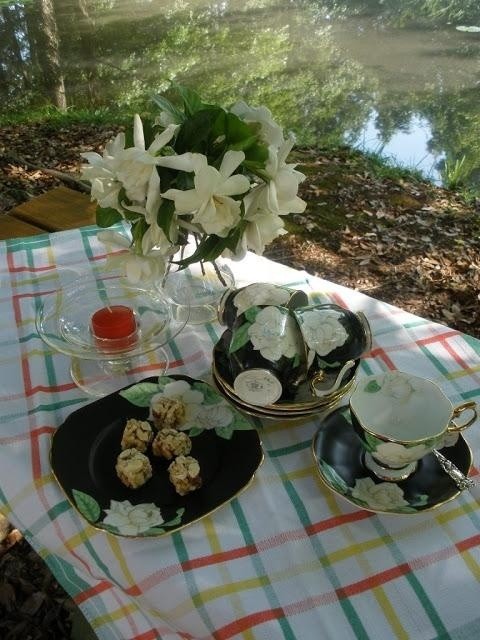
[
  {"xmin": 317, "ymin": 459, "xmax": 426, "ymax": 516},
  {"xmin": 124, "ymin": 284, "xmax": 349, "ymax": 438},
  {"xmin": 71, "ymin": 485, "xmax": 187, "ymax": 539},
  {"xmin": 361, "ymin": 374, "xmax": 424, "ymax": 422},
  {"xmin": 370, "ymin": 441, "xmax": 430, "ymax": 472},
  {"xmin": 80, "ymin": 77, "xmax": 307, "ymax": 285}
]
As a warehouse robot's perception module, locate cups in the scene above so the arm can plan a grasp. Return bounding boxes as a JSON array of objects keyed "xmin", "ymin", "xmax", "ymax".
[
  {"xmin": 218, "ymin": 282, "xmax": 307, "ymax": 326},
  {"xmin": 350, "ymin": 374, "xmax": 479, "ymax": 484},
  {"xmin": 292, "ymin": 303, "xmax": 372, "ymax": 396},
  {"xmin": 229, "ymin": 304, "xmax": 307, "ymax": 407}
]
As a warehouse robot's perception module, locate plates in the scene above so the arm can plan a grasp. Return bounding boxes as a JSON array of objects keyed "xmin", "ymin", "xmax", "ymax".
[
  {"xmin": 313, "ymin": 401, "xmax": 473, "ymax": 510},
  {"xmin": 49, "ymin": 373, "xmax": 263, "ymax": 536},
  {"xmin": 212, "ymin": 340, "xmax": 359, "ymax": 420}
]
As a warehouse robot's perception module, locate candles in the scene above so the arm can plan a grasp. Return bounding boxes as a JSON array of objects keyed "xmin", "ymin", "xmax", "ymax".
[{"xmin": 91, "ymin": 307, "xmax": 139, "ymax": 356}]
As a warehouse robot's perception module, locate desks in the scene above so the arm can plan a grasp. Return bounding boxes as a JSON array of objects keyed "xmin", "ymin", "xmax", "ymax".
[{"xmin": 0, "ymin": 187, "xmax": 479, "ymax": 640}]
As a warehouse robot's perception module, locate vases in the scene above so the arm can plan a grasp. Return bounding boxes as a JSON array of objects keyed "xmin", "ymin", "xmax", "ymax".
[{"xmin": 152, "ymin": 228, "xmax": 235, "ymax": 326}]
